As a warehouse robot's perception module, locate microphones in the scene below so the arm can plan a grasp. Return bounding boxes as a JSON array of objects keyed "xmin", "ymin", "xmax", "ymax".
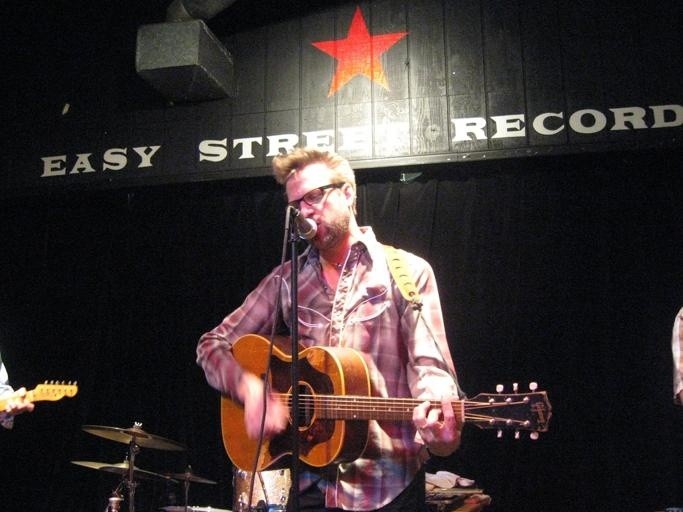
[{"xmin": 291, "ymin": 208, "xmax": 317, "ymax": 240}]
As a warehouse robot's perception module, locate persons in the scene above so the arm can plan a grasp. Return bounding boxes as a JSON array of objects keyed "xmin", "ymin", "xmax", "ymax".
[
  {"xmin": 666, "ymin": 306, "xmax": 683, "ymax": 512},
  {"xmin": 195, "ymin": 146, "xmax": 463, "ymax": 511},
  {"xmin": 0, "ymin": 351, "xmax": 35, "ymax": 430}
]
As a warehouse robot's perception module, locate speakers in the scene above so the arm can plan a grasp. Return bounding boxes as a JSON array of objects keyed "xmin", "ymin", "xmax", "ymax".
[{"xmin": 136, "ymin": 19, "xmax": 234, "ymax": 104}]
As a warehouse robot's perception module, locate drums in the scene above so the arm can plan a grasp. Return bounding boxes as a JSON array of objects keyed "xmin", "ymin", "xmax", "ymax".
[{"xmin": 232, "ymin": 464, "xmax": 293, "ymax": 511}]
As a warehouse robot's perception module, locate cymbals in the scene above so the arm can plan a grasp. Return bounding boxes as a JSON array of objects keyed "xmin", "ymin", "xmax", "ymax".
[
  {"xmin": 163, "ymin": 474, "xmax": 216, "ymax": 486},
  {"xmin": 68, "ymin": 461, "xmax": 180, "ymax": 486},
  {"xmin": 158, "ymin": 506, "xmax": 234, "ymax": 512},
  {"xmin": 81, "ymin": 425, "xmax": 186, "ymax": 451}
]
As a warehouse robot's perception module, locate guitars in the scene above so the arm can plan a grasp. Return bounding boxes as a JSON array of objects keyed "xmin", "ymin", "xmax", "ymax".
[{"xmin": 220, "ymin": 332, "xmax": 553, "ymax": 472}]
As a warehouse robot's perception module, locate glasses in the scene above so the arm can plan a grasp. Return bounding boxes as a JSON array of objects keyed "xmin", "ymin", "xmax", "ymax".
[{"xmin": 284, "ymin": 183, "xmax": 344, "ymax": 214}]
